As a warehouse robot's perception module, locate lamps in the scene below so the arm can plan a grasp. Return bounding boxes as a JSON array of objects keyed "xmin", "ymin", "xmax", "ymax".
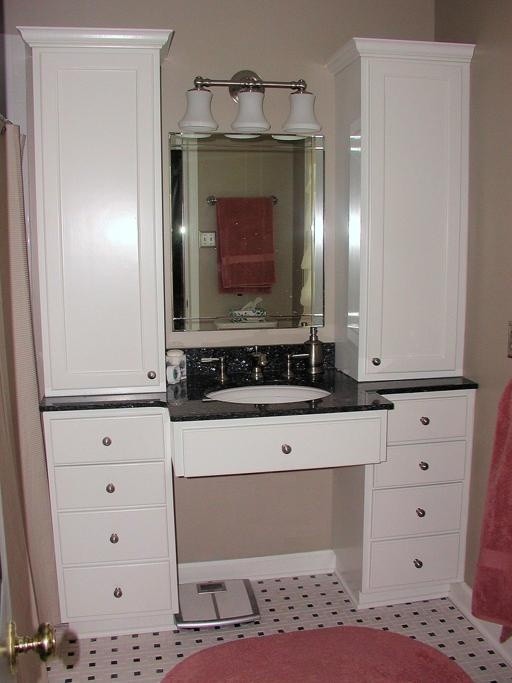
[{"xmin": 176, "ymin": 68, "xmax": 321, "ymax": 134}]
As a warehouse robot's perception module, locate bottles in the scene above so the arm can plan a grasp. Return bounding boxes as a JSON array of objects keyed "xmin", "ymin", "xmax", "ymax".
[{"xmin": 167, "ymin": 349, "xmax": 189, "ymax": 384}]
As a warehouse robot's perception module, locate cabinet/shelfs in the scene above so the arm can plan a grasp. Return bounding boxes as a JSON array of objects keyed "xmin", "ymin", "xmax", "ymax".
[
  {"xmin": 324, "ymin": 36, "xmax": 477, "ymax": 384},
  {"xmin": 171, "ymin": 407, "xmax": 389, "ymax": 478},
  {"xmin": 331, "ymin": 388, "xmax": 476, "ymax": 611},
  {"xmin": 40, "ymin": 405, "xmax": 181, "ymax": 643},
  {"xmin": 17, "ymin": 24, "xmax": 174, "ymax": 399}
]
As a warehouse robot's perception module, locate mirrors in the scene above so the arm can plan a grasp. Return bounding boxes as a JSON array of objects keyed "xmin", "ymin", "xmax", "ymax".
[{"xmin": 168, "ymin": 130, "xmax": 326, "ymax": 333}]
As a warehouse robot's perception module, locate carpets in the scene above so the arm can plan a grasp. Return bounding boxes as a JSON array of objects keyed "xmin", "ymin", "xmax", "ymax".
[{"xmin": 159, "ymin": 625, "xmax": 475, "ymax": 682}]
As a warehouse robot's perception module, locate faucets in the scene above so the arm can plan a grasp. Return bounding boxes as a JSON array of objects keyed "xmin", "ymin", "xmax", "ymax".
[{"xmin": 250, "ymin": 346, "xmax": 268, "ymax": 382}]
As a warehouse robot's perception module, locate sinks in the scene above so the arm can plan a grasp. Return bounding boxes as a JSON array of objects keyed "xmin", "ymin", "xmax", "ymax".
[{"xmin": 204, "ymin": 384, "xmax": 332, "ymax": 405}]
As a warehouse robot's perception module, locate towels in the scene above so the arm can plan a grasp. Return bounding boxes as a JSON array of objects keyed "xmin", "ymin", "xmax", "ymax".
[
  {"xmin": 471, "ymin": 377, "xmax": 511, "ymax": 644},
  {"xmin": 216, "ymin": 195, "xmax": 278, "ymax": 295}
]
